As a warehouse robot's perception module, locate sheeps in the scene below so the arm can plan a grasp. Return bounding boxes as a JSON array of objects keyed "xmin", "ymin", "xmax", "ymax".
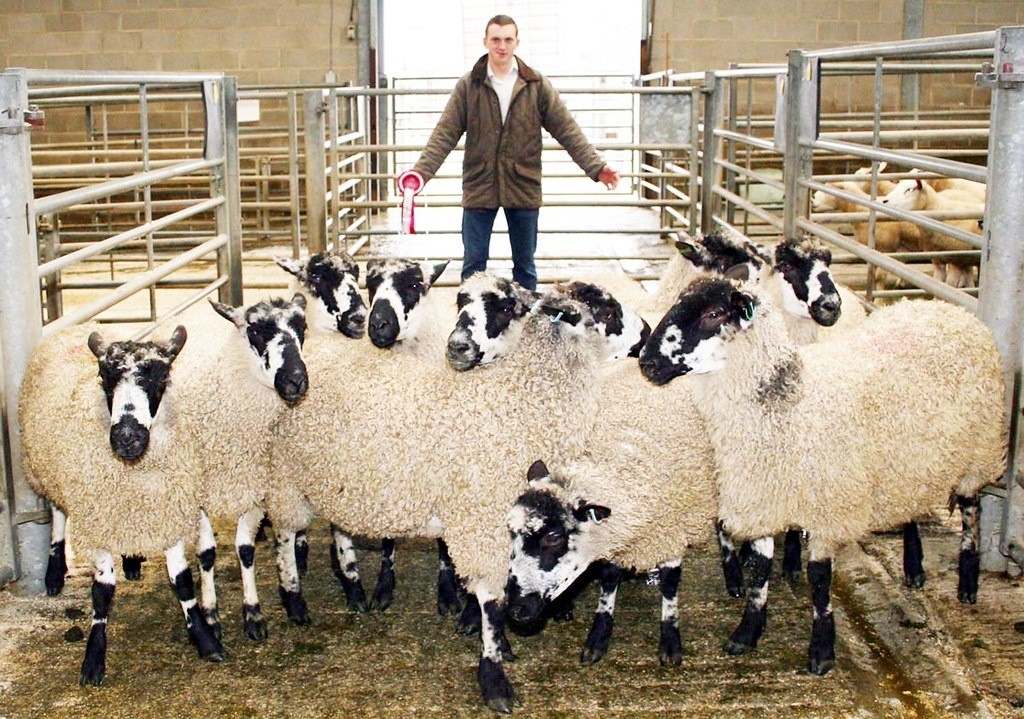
[
  {"xmin": 807, "ymin": 159, "xmax": 988, "ymax": 306},
  {"xmin": 14, "ymin": 226, "xmax": 1010, "ymax": 717}
]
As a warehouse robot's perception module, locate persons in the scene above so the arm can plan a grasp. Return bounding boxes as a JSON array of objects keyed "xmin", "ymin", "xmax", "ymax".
[{"xmin": 398, "ymin": 14, "xmax": 618, "ymax": 293}]
